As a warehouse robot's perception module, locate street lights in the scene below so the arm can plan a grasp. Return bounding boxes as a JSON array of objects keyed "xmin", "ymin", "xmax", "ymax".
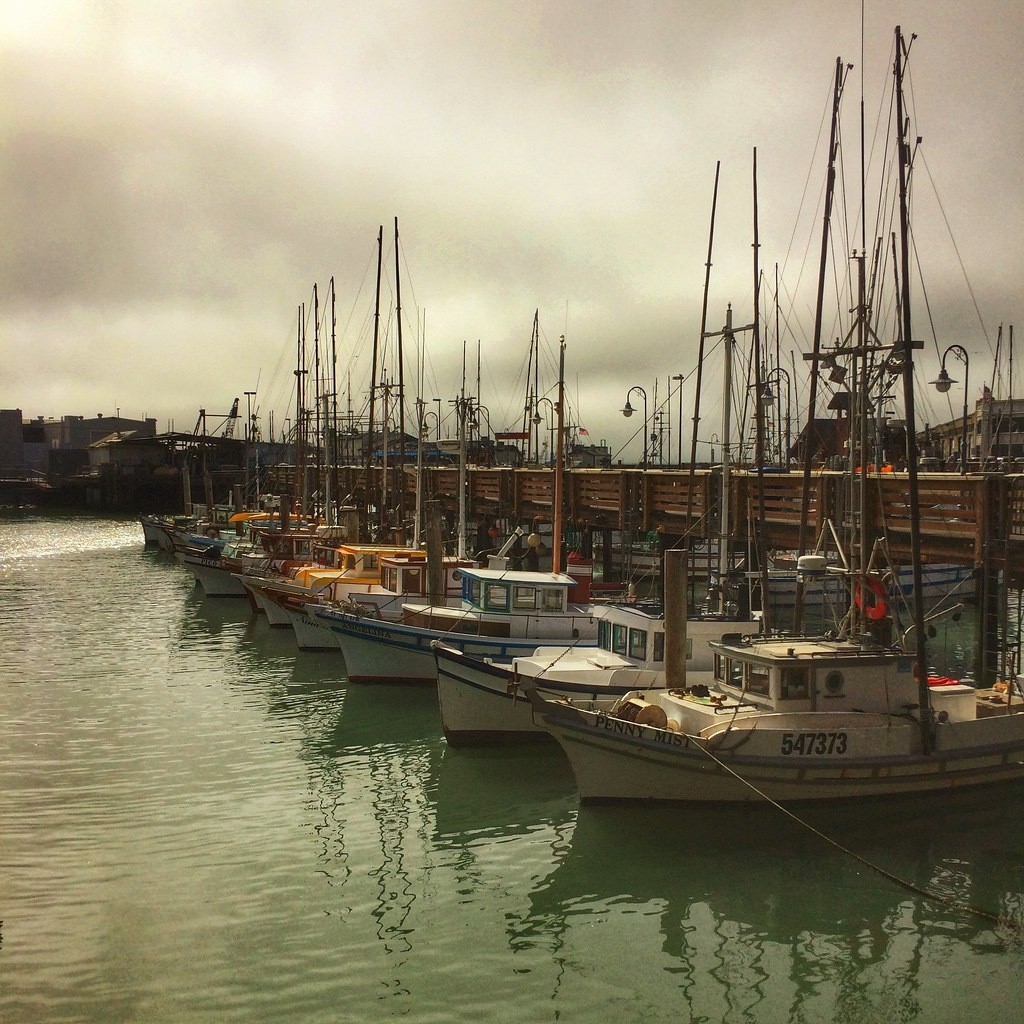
[
  {"xmin": 672, "ymin": 374, "xmax": 685, "ymax": 469},
  {"xmin": 244, "ymin": 391, "xmax": 257, "ymax": 440},
  {"xmin": 925, "ymin": 345, "xmax": 972, "ymax": 476},
  {"xmin": 620, "ymin": 386, "xmax": 648, "ymax": 472},
  {"xmin": 529, "ymin": 397, "xmax": 555, "ymax": 470},
  {"xmin": 469, "ymin": 406, "xmax": 492, "ymax": 468},
  {"xmin": 422, "ymin": 412, "xmax": 440, "ymax": 468},
  {"xmin": 431, "ymin": 398, "xmax": 441, "ymax": 442}
]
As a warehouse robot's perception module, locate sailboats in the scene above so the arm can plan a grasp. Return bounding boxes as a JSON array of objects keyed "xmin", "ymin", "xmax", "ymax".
[{"xmin": 138, "ymin": 0, "xmax": 1024, "ymax": 808}]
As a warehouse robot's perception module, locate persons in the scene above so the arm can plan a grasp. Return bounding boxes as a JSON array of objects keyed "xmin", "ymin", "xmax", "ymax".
[{"xmin": 945, "ymin": 451, "xmax": 961, "ymax": 473}]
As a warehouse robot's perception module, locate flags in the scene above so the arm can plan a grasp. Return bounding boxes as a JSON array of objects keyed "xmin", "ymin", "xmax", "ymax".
[{"xmin": 579, "ymin": 428, "xmax": 589, "ymax": 436}]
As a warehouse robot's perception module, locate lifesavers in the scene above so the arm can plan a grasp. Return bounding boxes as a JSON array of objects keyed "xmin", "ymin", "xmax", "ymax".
[
  {"xmin": 850, "ymin": 577, "xmax": 887, "ymax": 618},
  {"xmin": 203, "ymin": 526, "xmax": 219, "ymax": 539}
]
